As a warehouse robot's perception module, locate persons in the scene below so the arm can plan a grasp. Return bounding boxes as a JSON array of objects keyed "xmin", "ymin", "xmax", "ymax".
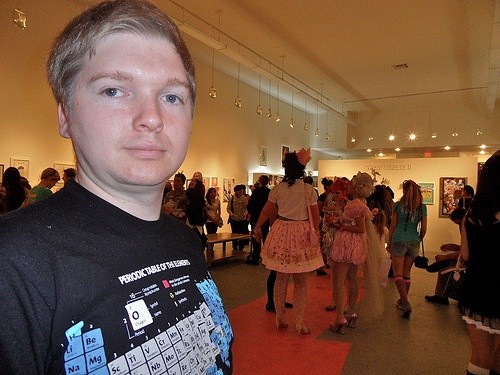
[
  {"xmin": 0, "ymin": 0, "xmax": 234, "ymax": 375},
  {"xmin": 0, "ymin": 149, "xmax": 500, "ymax": 375}
]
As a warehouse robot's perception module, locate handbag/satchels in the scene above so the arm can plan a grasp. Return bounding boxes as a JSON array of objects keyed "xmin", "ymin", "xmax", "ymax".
[
  {"xmin": 215, "ymin": 214, "xmax": 223, "ymax": 227},
  {"xmin": 414, "ymin": 239, "xmax": 429, "ymax": 269},
  {"xmin": 309, "ymin": 229, "xmax": 318, "ymax": 246}
]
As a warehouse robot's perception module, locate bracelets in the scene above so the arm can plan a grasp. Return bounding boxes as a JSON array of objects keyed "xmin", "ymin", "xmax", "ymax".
[{"xmin": 339, "ymin": 225, "xmax": 345, "ymax": 232}]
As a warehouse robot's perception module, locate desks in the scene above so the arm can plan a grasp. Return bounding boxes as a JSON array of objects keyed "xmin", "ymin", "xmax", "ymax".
[{"xmin": 204, "ymin": 232, "xmax": 252, "ymax": 267}]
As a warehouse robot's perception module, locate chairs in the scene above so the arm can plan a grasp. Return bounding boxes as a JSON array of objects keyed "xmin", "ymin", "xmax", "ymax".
[{"xmin": 439, "ymin": 254, "xmax": 469, "ymax": 299}]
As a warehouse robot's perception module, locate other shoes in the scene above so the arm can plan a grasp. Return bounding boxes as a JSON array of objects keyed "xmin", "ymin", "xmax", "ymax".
[
  {"xmin": 426, "ymin": 259, "xmax": 457, "ymax": 273},
  {"xmin": 402, "ymin": 305, "xmax": 412, "ymax": 319},
  {"xmin": 425, "ymin": 295, "xmax": 449, "ymax": 305},
  {"xmin": 265, "ymin": 302, "xmax": 293, "ymax": 313},
  {"xmin": 324, "ymin": 264, "xmax": 331, "ymax": 268},
  {"xmin": 316, "ymin": 270, "xmax": 328, "ymax": 276},
  {"xmin": 396, "ymin": 299, "xmax": 404, "ymax": 309},
  {"xmin": 326, "ymin": 303, "xmax": 337, "ymax": 311},
  {"xmin": 246, "ymin": 259, "xmax": 259, "ymax": 265}
]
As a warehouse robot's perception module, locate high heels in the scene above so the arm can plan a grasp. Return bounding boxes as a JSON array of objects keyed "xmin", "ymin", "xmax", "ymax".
[
  {"xmin": 276, "ymin": 320, "xmax": 288, "ymax": 330},
  {"xmin": 329, "ymin": 318, "xmax": 347, "ymax": 334},
  {"xmin": 297, "ymin": 324, "xmax": 310, "ymax": 335},
  {"xmin": 344, "ymin": 312, "xmax": 359, "ymax": 329}
]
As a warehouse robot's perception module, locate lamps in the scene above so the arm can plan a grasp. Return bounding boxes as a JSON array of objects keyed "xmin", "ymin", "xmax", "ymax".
[
  {"xmin": 235, "ymin": 43, "xmax": 242, "ymax": 108},
  {"xmin": 314, "ymin": 93, "xmax": 320, "ymax": 137},
  {"xmin": 265, "ymin": 62, "xmax": 273, "ymax": 119},
  {"xmin": 303, "ymin": 86, "xmax": 308, "ymax": 132},
  {"xmin": 256, "ymin": 57, "xmax": 263, "ymax": 116},
  {"xmin": 208, "ymin": 28, "xmax": 216, "ymax": 98},
  {"xmin": 325, "ymin": 99, "xmax": 330, "ymax": 140},
  {"xmin": 274, "ymin": 76, "xmax": 280, "ymax": 122},
  {"xmin": 288, "ymin": 77, "xmax": 295, "ymax": 128}
]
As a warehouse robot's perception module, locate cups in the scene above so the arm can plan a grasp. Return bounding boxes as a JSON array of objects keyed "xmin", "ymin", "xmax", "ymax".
[{"xmin": 327, "ymin": 210, "xmax": 340, "ymax": 225}]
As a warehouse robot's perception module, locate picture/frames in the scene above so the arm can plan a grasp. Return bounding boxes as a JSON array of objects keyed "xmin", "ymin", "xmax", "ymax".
[
  {"xmin": 54, "ymin": 162, "xmax": 75, "ymax": 185},
  {"xmin": 0, "ymin": 165, "xmax": 4, "ymax": 185},
  {"xmin": 258, "ymin": 143, "xmax": 268, "ymax": 167},
  {"xmin": 280, "ymin": 144, "xmax": 290, "ymax": 167},
  {"xmin": 418, "ymin": 182, "xmax": 435, "ymax": 205},
  {"xmin": 438, "ymin": 177, "xmax": 468, "ymax": 218},
  {"xmin": 10, "ymin": 158, "xmax": 30, "ymax": 182}
]
